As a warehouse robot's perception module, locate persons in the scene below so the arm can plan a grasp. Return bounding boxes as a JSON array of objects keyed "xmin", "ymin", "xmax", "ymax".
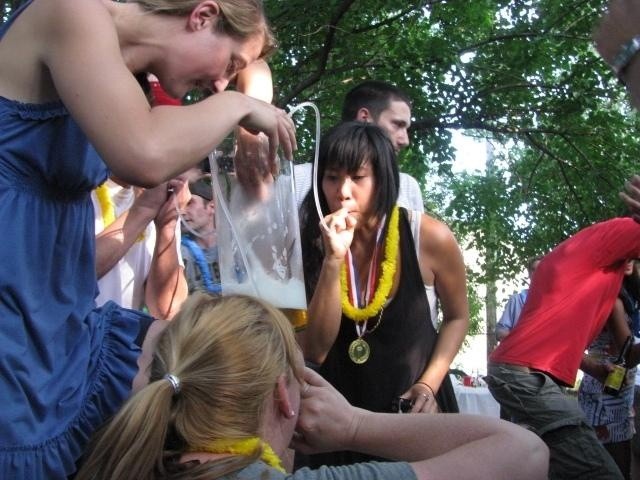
[
  {"xmin": 90, "ymin": 172, "xmax": 190, "ymax": 318},
  {"xmin": 593, "ymin": 0, "xmax": 640, "ymax": 215},
  {"xmin": 0, "ymin": 0, "xmax": 298, "ymax": 480},
  {"xmin": 73, "ymin": 290, "xmax": 551, "ymax": 480},
  {"xmin": 178, "ymin": 174, "xmax": 246, "ymax": 297},
  {"xmin": 480, "ymin": 211, "xmax": 640, "ymax": 480},
  {"xmin": 577, "ymin": 257, "xmax": 639, "ymax": 480},
  {"xmin": 492, "ymin": 255, "xmax": 548, "ymax": 340},
  {"xmin": 294, "ymin": 78, "xmax": 424, "ymax": 217}
]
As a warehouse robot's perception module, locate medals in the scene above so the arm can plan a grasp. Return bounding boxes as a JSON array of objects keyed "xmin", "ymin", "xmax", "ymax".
[{"xmin": 348, "ymin": 335, "xmax": 372, "ymax": 366}]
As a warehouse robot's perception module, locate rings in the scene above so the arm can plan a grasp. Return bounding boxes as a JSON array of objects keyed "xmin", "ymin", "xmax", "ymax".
[{"xmin": 420, "ymin": 393, "xmax": 427, "ymax": 401}]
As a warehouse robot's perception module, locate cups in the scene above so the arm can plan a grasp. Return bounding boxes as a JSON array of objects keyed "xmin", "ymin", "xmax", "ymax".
[{"xmin": 208, "ymin": 131, "xmax": 311, "ymax": 336}]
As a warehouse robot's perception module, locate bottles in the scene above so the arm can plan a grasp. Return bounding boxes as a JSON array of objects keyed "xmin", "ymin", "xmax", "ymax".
[{"xmin": 601, "ymin": 333, "xmax": 637, "ymax": 401}]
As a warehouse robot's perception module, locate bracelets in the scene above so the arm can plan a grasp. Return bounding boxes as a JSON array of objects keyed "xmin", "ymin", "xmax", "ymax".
[{"xmin": 416, "ymin": 379, "xmax": 436, "ymax": 399}]
